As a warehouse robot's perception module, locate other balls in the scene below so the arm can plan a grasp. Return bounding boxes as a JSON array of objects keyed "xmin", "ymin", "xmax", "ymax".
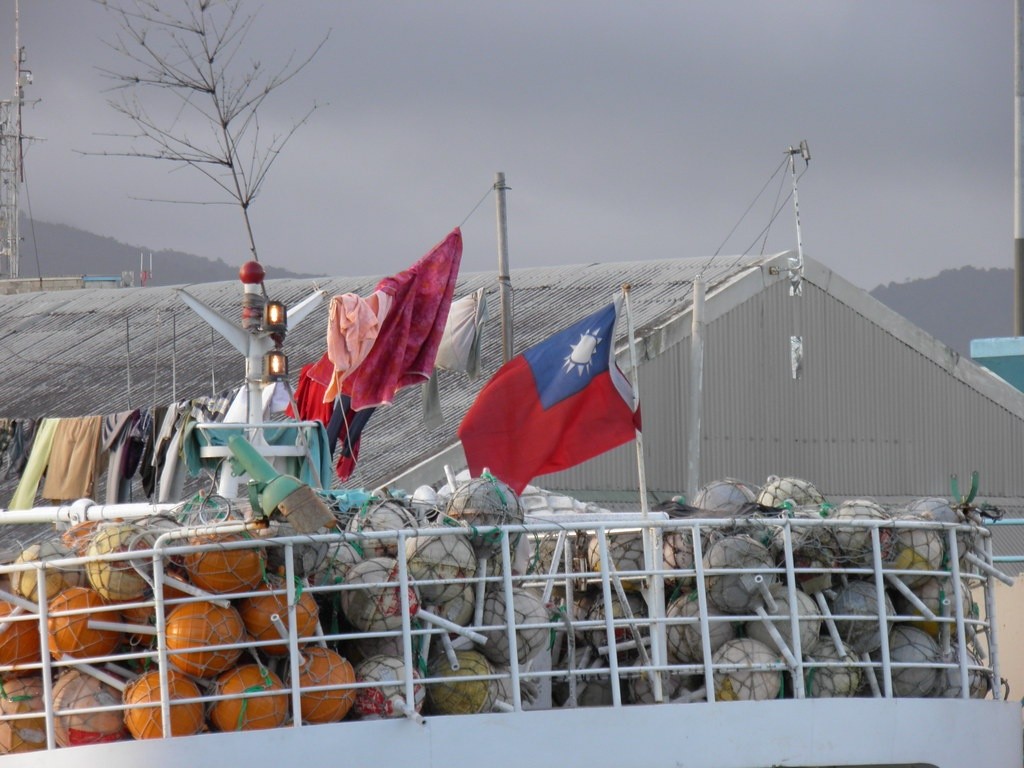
[{"xmin": 1, "ymin": 470, "xmax": 994, "ymax": 754}]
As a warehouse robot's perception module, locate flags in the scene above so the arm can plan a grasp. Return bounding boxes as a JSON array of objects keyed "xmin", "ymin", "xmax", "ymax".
[{"xmin": 456, "ymin": 288, "xmax": 645, "ymax": 504}]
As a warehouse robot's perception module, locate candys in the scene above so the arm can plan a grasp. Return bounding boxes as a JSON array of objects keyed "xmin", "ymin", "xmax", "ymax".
[{"xmin": 1, "ymin": 462, "xmax": 989, "ymax": 758}]
